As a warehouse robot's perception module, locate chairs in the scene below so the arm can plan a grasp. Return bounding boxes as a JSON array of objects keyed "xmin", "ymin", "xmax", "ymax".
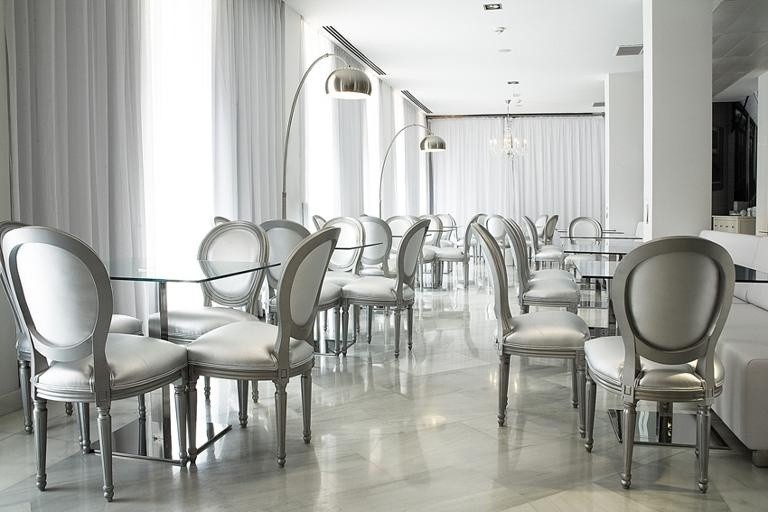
[
  {"xmin": 339, "ymin": 219, "xmax": 432, "ymax": 358},
  {"xmin": 261, "ymin": 219, "xmax": 342, "ymax": 356},
  {"xmin": 208, "ymin": 210, "xmax": 736, "ymax": 290},
  {"xmin": 467, "ymin": 220, "xmax": 591, "ymax": 438},
  {"xmin": 0, "ymin": 220, "xmax": 143, "ymax": 449},
  {"xmin": 147, "ymin": 219, "xmax": 269, "ymax": 428},
  {"xmin": 185, "ymin": 227, "xmax": 342, "ymax": 468},
  {"xmin": 0, "ymin": 225, "xmax": 190, "ymax": 503},
  {"xmin": 584, "ymin": 236, "xmax": 740, "ymax": 495}
]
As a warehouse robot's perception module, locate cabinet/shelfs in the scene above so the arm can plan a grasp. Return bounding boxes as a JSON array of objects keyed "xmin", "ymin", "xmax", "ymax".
[{"xmin": 712, "ymin": 214, "xmax": 756, "ymax": 237}]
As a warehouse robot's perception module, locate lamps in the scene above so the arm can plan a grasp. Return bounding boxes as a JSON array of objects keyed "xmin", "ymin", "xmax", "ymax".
[
  {"xmin": 281, "ymin": 52, "xmax": 371, "ymax": 222},
  {"xmin": 484, "ymin": 97, "xmax": 530, "ymax": 161},
  {"xmin": 373, "ymin": 122, "xmax": 446, "ymax": 219}
]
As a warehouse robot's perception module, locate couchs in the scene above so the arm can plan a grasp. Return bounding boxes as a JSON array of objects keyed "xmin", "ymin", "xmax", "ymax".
[{"xmin": 699, "ymin": 229, "xmax": 766, "ymax": 470}]
